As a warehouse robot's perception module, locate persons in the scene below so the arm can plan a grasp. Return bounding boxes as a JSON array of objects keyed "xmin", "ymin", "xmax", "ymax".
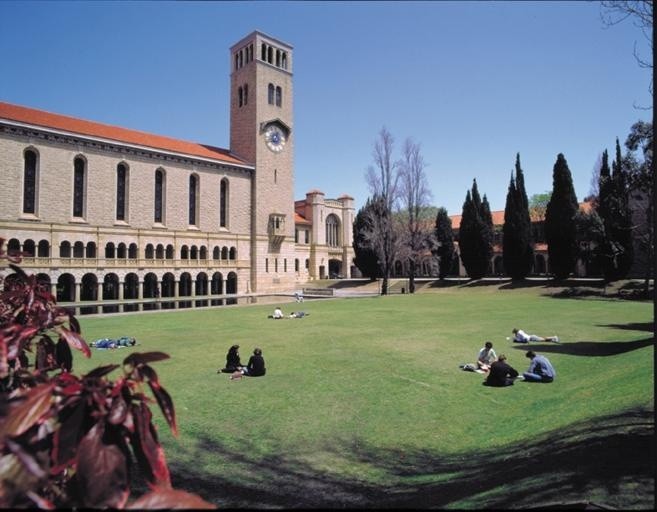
[
  {"xmin": 290, "ymin": 312, "xmax": 305, "ymax": 319},
  {"xmin": 523, "ymin": 350, "xmax": 557, "ymax": 383},
  {"xmin": 477, "ymin": 342, "xmax": 498, "ymax": 371},
  {"xmin": 486, "ymin": 355, "xmax": 519, "ymax": 387},
  {"xmin": 115, "ymin": 337, "xmax": 137, "ymax": 347},
  {"xmin": 242, "ymin": 347, "xmax": 268, "ymax": 376},
  {"xmin": 90, "ymin": 337, "xmax": 117, "ymax": 348},
  {"xmin": 273, "ymin": 307, "xmax": 284, "ymax": 319},
  {"xmin": 217, "ymin": 345, "xmax": 242, "ymax": 373},
  {"xmin": 511, "ymin": 327, "xmax": 559, "ymax": 343}
]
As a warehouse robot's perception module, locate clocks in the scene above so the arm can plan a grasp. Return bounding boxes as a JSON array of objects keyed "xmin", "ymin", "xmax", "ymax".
[{"xmin": 264, "ymin": 125, "xmax": 285, "ymax": 153}]
